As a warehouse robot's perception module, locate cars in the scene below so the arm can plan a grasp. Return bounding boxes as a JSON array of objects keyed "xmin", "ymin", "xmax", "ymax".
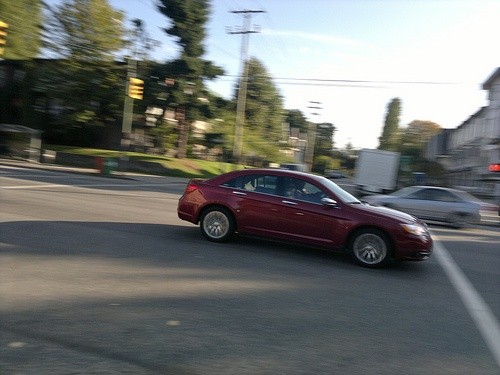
[
  {"xmin": 176, "ymin": 168, "xmax": 433, "ymax": 268},
  {"xmin": 363, "ymin": 185, "xmax": 483, "ymax": 230}
]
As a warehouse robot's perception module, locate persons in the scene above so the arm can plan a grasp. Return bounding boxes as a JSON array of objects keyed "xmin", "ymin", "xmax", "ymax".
[{"xmin": 288, "ymin": 177, "xmax": 324, "ymax": 202}]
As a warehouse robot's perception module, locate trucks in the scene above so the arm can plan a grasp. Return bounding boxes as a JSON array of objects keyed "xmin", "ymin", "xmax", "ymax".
[{"xmin": 353, "ymin": 149, "xmax": 402, "ymax": 197}]
